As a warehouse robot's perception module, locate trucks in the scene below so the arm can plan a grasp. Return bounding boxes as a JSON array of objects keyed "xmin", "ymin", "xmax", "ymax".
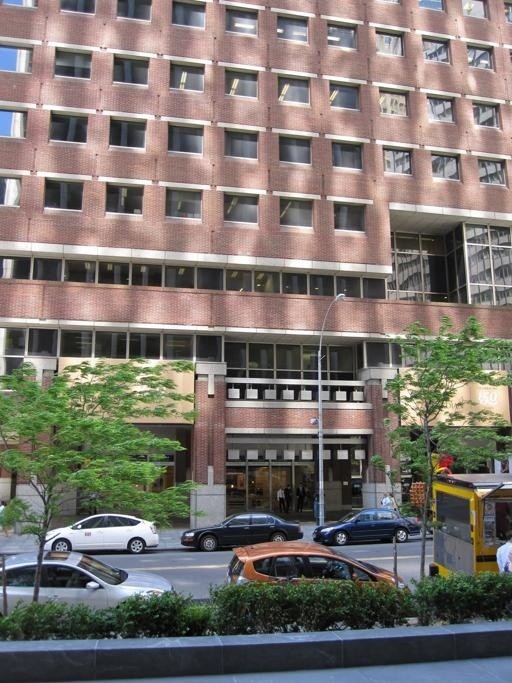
[{"xmin": 427, "ymin": 471, "xmax": 512, "ymax": 588}]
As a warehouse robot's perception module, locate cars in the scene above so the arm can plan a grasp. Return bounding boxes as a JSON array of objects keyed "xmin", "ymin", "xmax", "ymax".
[
  {"xmin": 0, "ymin": 553, "xmax": 175, "ymax": 620},
  {"xmin": 45, "ymin": 515, "xmax": 160, "ymax": 553},
  {"xmin": 180, "ymin": 513, "xmax": 303, "ymax": 553}
]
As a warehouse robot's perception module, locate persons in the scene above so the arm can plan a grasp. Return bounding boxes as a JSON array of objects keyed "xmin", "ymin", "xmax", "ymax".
[
  {"xmin": 285, "ymin": 484, "xmax": 292, "ymax": 512},
  {"xmin": 278, "ymin": 487, "xmax": 286, "ymax": 514},
  {"xmin": 388, "ymin": 493, "xmax": 399, "ymax": 511},
  {"xmin": 381, "ymin": 492, "xmax": 388, "ymax": 504},
  {"xmin": 496, "ymin": 530, "xmax": 512, "ymax": 574},
  {"xmin": 296, "ymin": 482, "xmax": 307, "ymax": 512},
  {"xmin": 0, "ymin": 500, "xmax": 10, "ymax": 538}
]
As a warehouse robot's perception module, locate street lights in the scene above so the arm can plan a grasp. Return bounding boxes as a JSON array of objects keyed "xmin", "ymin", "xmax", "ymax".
[{"xmin": 311, "ymin": 293, "xmax": 346, "ymax": 529}]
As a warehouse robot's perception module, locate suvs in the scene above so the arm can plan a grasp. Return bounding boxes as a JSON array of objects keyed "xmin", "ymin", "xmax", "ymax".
[
  {"xmin": 224, "ymin": 540, "xmax": 408, "ymax": 602},
  {"xmin": 314, "ymin": 508, "xmax": 421, "ymax": 545}
]
